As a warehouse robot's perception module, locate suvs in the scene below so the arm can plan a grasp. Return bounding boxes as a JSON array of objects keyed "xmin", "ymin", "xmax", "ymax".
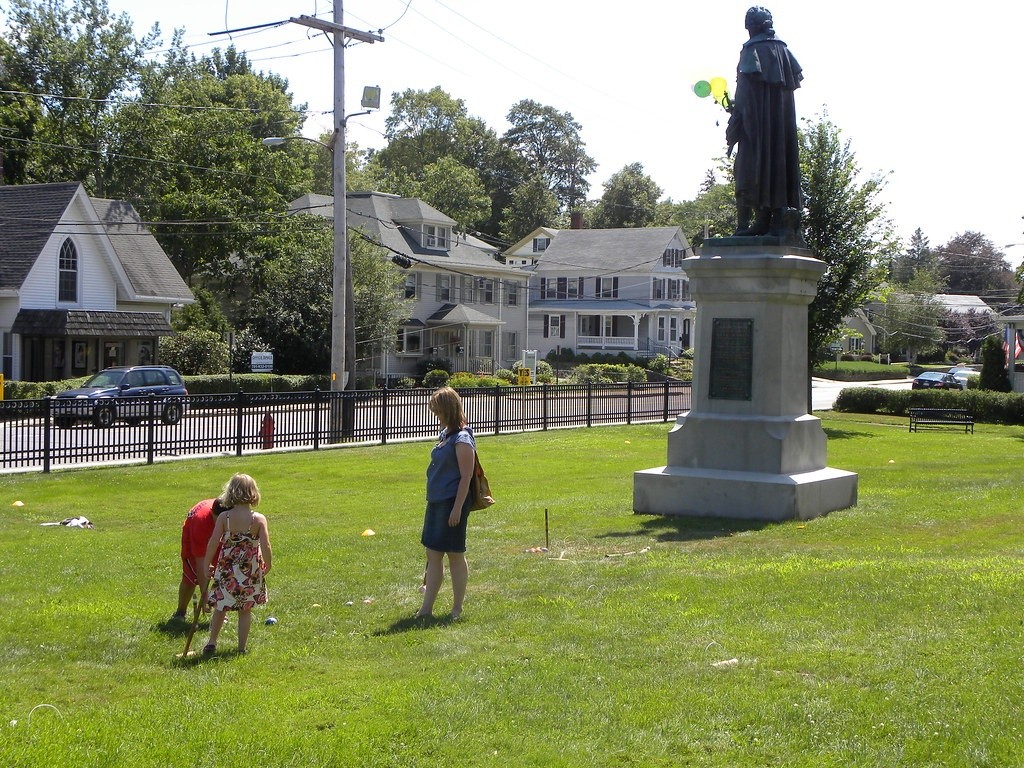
[{"xmin": 44, "ymin": 364, "xmax": 190, "ymax": 429}]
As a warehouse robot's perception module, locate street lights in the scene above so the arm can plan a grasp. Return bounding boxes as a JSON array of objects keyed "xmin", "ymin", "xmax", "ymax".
[{"xmin": 262, "ymin": 84, "xmax": 383, "ymax": 440}]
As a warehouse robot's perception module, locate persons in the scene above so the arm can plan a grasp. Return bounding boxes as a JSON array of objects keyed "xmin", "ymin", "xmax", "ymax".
[
  {"xmin": 172, "ymin": 495, "xmax": 234, "ymax": 623},
  {"xmin": 413, "ymin": 386, "xmax": 477, "ymax": 620},
  {"xmin": 201, "ymin": 473, "xmax": 273, "ymax": 651}
]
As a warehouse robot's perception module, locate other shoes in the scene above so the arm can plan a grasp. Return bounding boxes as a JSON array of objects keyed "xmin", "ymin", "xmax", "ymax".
[
  {"xmin": 236, "ymin": 650, "xmax": 249, "ymax": 655},
  {"xmin": 204, "ymin": 643, "xmax": 216, "ymax": 652}
]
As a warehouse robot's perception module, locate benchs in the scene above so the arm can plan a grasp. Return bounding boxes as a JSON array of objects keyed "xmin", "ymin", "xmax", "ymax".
[{"xmin": 908, "ymin": 408, "xmax": 975, "ymax": 435}]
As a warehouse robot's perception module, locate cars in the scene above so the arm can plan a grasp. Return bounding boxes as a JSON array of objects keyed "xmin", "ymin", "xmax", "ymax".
[
  {"xmin": 912, "ymin": 371, "xmax": 963, "ymax": 392},
  {"xmin": 947, "ymin": 367, "xmax": 977, "ymax": 375},
  {"xmin": 952, "ymin": 371, "xmax": 981, "ymax": 390}
]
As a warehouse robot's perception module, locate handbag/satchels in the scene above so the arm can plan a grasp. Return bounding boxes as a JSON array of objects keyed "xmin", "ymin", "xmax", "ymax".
[{"xmin": 467, "ymin": 449, "xmax": 496, "ymax": 511}]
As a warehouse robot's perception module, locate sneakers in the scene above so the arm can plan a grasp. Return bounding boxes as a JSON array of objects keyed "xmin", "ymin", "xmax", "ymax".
[{"xmin": 173, "ymin": 609, "xmax": 186, "ymax": 622}]
